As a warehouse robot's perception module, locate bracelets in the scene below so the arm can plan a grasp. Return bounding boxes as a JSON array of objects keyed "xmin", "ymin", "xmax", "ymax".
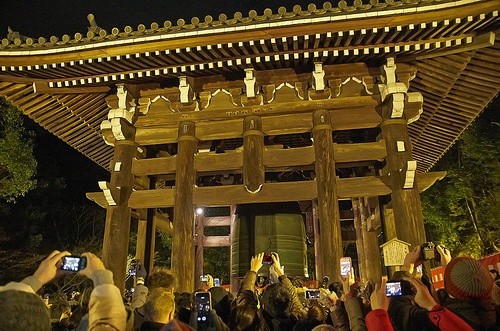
[{"xmin": 343, "ymin": 293, "xmax": 353, "ymax": 300}]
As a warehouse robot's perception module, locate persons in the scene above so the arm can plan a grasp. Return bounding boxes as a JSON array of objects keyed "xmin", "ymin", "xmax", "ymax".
[
  {"xmin": 0, "ymin": 250, "xmax": 234, "ymax": 331},
  {"xmin": 401, "ymin": 244, "xmax": 500, "ymax": 331},
  {"xmin": 230, "ymin": 252, "xmax": 475, "ymax": 331}
]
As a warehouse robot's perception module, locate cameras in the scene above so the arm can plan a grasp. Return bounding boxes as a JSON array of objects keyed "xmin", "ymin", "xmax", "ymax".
[
  {"xmin": 385, "ymin": 279, "xmax": 410, "ymax": 297},
  {"xmin": 262, "ymin": 252, "xmax": 273, "ymax": 264},
  {"xmin": 419, "ymin": 242, "xmax": 445, "ymax": 261},
  {"xmin": 59, "ymin": 256, "xmax": 86, "ymax": 272},
  {"xmin": 200, "ymin": 276, "xmax": 208, "ymax": 281}
]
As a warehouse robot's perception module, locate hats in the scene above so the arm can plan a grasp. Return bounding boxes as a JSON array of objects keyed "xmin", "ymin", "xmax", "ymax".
[
  {"xmin": 444, "ymin": 256, "xmax": 494, "ymax": 299},
  {"xmin": 0, "ymin": 290, "xmax": 51, "ymax": 331},
  {"xmin": 131, "ymin": 285, "xmax": 148, "ymax": 308}
]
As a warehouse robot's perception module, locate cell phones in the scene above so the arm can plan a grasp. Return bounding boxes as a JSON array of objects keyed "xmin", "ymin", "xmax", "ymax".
[
  {"xmin": 214, "ymin": 278, "xmax": 219, "ymax": 287},
  {"xmin": 195, "ymin": 292, "xmax": 212, "ymax": 331},
  {"xmin": 304, "ymin": 290, "xmax": 323, "ymax": 300},
  {"xmin": 339, "ymin": 257, "xmax": 352, "ymax": 278}
]
